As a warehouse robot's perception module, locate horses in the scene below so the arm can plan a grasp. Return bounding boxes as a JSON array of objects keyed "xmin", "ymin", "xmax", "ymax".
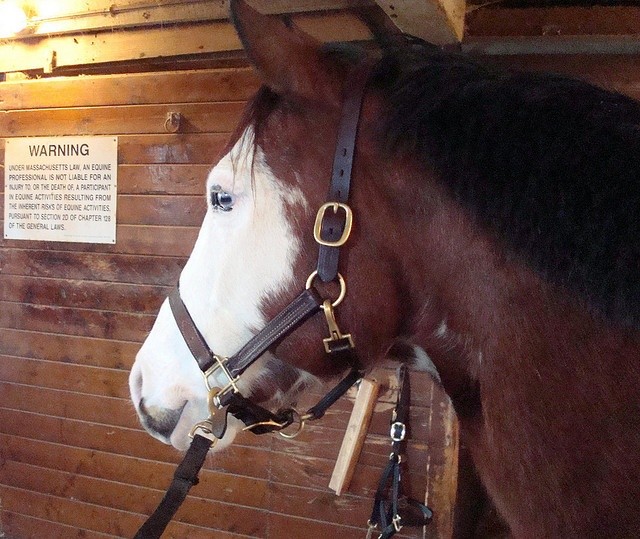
[{"xmin": 128, "ymin": 0, "xmax": 639, "ymax": 539}]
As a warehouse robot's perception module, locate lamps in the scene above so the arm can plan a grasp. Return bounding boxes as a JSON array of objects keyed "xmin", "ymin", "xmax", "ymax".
[{"xmin": 0, "ymin": 1, "xmax": 33, "ymax": 39}]
